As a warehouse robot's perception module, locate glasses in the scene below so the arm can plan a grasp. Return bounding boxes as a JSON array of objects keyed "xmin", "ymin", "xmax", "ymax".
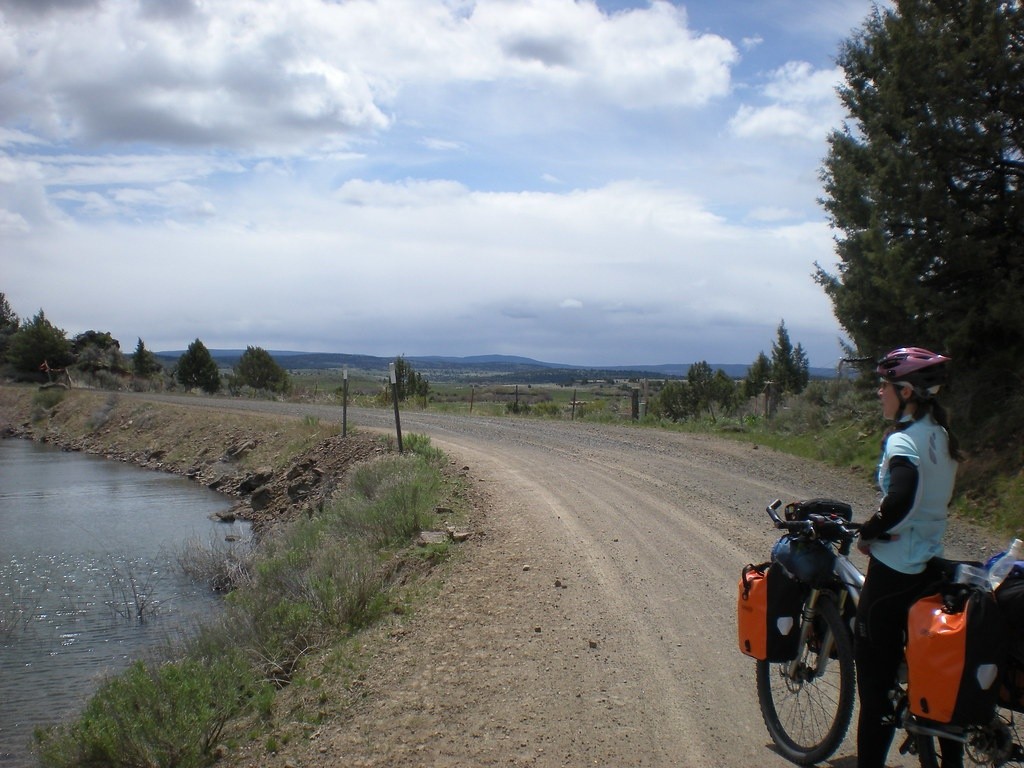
[{"xmin": 879, "ymin": 377, "xmax": 913, "ymax": 393}]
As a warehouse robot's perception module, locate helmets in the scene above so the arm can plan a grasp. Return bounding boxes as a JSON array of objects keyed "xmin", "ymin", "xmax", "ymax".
[{"xmin": 877, "ymin": 347, "xmax": 952, "ymax": 382}]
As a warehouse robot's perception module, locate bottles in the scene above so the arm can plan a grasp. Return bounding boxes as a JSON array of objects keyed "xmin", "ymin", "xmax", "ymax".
[
  {"xmin": 989, "ymin": 538, "xmax": 1024, "ymax": 591},
  {"xmin": 957, "ymin": 565, "xmax": 991, "ymax": 592}
]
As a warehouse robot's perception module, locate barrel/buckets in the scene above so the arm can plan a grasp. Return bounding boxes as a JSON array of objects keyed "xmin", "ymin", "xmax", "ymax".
[
  {"xmin": 738, "ymin": 561, "xmax": 773, "ymax": 659},
  {"xmin": 905, "ymin": 593, "xmax": 968, "ymax": 723}
]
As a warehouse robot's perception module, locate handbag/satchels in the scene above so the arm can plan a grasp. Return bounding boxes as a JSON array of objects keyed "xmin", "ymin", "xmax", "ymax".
[
  {"xmin": 906, "ymin": 554, "xmax": 1024, "ymax": 728},
  {"xmin": 738, "ymin": 548, "xmax": 839, "ymax": 664}
]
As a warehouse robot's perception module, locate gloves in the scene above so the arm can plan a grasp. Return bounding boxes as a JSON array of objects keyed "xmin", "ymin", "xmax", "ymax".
[{"xmin": 857, "ymin": 536, "xmax": 870, "ymax": 555}]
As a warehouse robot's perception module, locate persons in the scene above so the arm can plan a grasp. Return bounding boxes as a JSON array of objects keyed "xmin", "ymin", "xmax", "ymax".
[{"xmin": 853, "ymin": 347, "xmax": 964, "ymax": 768}]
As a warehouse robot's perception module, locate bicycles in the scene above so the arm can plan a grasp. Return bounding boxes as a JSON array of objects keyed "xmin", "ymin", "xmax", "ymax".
[{"xmin": 755, "ymin": 498, "xmax": 1024, "ymax": 768}]
